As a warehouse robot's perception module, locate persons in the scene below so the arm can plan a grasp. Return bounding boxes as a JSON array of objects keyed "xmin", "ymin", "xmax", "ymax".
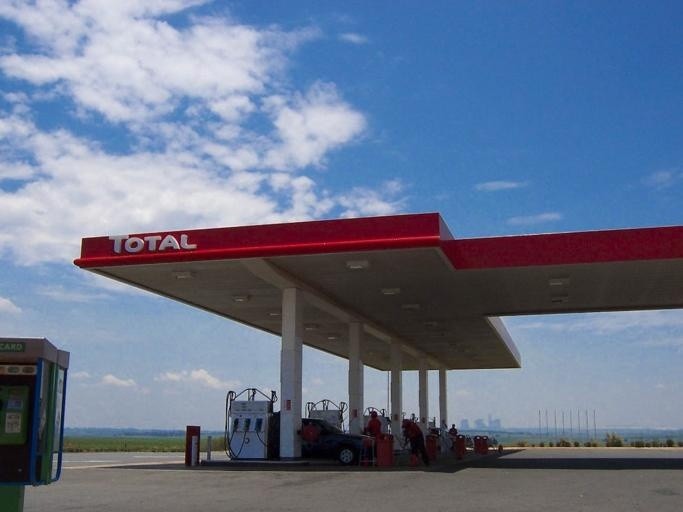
[
  {"xmin": 367, "ymin": 411, "xmax": 381, "ymax": 458},
  {"xmin": 402, "ymin": 419, "xmax": 430, "ymax": 465},
  {"xmin": 449, "ymin": 424, "xmax": 457, "ymax": 450}
]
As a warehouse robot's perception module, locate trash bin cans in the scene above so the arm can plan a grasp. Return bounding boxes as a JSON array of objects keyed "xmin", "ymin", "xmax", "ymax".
[
  {"xmin": 376, "ymin": 433, "xmax": 394, "ymax": 466},
  {"xmin": 456, "ymin": 435, "xmax": 465, "ymax": 455},
  {"xmin": 473, "ymin": 436, "xmax": 488, "ymax": 455},
  {"xmin": 426, "ymin": 434, "xmax": 438, "ymax": 465}
]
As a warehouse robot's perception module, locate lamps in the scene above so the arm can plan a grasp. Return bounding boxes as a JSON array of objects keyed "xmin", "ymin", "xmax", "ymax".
[
  {"xmin": 403, "ymin": 304, "xmax": 423, "ymax": 312},
  {"xmin": 550, "ymin": 277, "xmax": 569, "ymax": 288},
  {"xmin": 347, "ymin": 261, "xmax": 376, "ymax": 272},
  {"xmin": 303, "ymin": 323, "xmax": 319, "ymax": 332},
  {"xmin": 175, "ymin": 270, "xmax": 198, "ymax": 281},
  {"xmin": 380, "ymin": 287, "xmax": 402, "ymax": 296},
  {"xmin": 267, "ymin": 310, "xmax": 282, "ymax": 319},
  {"xmin": 550, "ymin": 296, "xmax": 568, "ymax": 304},
  {"xmin": 327, "ymin": 333, "xmax": 341, "ymax": 341},
  {"xmin": 232, "ymin": 295, "xmax": 250, "ymax": 304},
  {"xmin": 423, "ymin": 321, "xmax": 441, "ymax": 328}
]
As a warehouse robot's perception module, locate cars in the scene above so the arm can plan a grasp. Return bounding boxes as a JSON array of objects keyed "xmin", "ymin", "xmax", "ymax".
[{"xmin": 301, "ymin": 417, "xmax": 375, "ymax": 465}]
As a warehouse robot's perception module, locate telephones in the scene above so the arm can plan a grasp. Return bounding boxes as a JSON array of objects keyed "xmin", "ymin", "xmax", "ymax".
[{"xmin": 0, "ymin": 385, "xmax": 30, "ymax": 445}]
